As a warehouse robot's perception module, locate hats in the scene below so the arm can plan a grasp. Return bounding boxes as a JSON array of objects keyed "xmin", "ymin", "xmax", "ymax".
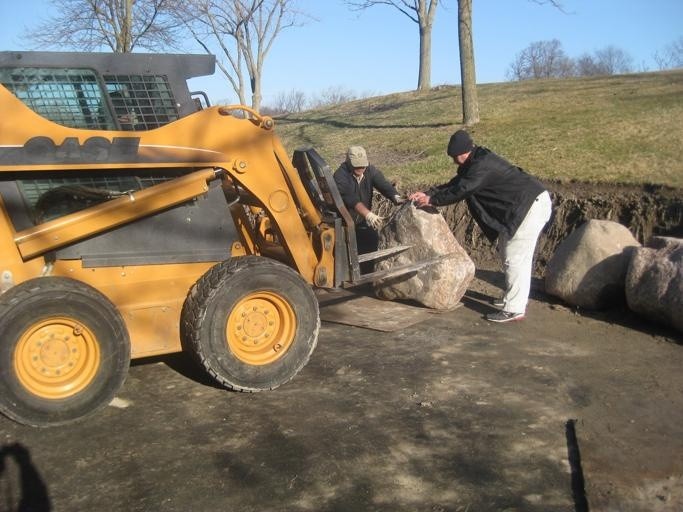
[
  {"xmin": 448, "ymin": 131, "xmax": 470, "ymax": 158},
  {"xmin": 347, "ymin": 146, "xmax": 369, "ymax": 168}
]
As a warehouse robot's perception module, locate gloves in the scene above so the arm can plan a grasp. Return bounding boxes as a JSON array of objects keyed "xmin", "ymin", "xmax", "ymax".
[{"xmin": 366, "ymin": 212, "xmax": 382, "ymax": 229}]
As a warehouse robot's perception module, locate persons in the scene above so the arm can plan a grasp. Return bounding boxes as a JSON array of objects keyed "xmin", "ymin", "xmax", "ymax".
[
  {"xmin": 333, "ymin": 146, "xmax": 407, "ymax": 232},
  {"xmin": 408, "ymin": 129, "xmax": 553, "ymax": 323}
]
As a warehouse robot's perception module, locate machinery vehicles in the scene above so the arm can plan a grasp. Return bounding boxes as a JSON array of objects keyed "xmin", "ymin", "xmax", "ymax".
[{"xmin": 0, "ymin": 48, "xmax": 451, "ymax": 433}]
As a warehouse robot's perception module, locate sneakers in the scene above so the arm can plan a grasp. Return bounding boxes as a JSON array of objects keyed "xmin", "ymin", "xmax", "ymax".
[
  {"xmin": 487, "ymin": 311, "xmax": 524, "ymax": 323},
  {"xmin": 493, "ymin": 298, "xmax": 505, "ymax": 306}
]
